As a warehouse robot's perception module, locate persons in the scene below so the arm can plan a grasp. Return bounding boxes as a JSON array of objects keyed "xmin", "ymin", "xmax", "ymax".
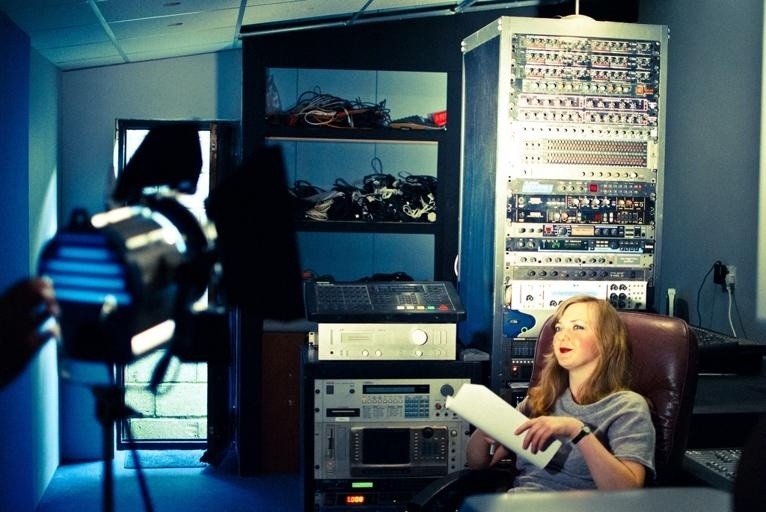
[
  {"xmin": 468, "ymin": 296, "xmax": 657, "ymax": 490},
  {"xmin": 0, "ymin": 277, "xmax": 62, "ymax": 394}
]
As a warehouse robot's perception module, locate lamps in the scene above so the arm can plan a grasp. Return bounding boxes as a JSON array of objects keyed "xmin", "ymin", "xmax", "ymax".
[{"xmin": 559, "ymin": 0, "xmax": 596, "ymax": 32}]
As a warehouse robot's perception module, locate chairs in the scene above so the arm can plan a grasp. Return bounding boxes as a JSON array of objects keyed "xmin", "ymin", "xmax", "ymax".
[{"xmin": 411, "ymin": 308, "xmax": 697, "ymax": 512}]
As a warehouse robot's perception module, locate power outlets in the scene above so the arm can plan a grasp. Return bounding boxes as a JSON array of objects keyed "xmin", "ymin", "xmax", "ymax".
[{"xmin": 718, "ymin": 264, "xmax": 736, "ymax": 291}]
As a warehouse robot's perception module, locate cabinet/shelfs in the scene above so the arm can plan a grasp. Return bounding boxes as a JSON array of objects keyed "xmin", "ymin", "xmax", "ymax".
[{"xmin": 236, "ymin": 0, "xmax": 461, "ymax": 471}]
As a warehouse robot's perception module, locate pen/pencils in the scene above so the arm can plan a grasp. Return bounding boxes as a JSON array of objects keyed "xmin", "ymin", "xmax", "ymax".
[{"xmin": 490, "ymin": 440, "xmax": 498, "ymax": 457}]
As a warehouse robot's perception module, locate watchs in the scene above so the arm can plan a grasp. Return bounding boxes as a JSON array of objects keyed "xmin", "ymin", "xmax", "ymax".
[{"xmin": 572, "ymin": 425, "xmax": 591, "ymax": 444}]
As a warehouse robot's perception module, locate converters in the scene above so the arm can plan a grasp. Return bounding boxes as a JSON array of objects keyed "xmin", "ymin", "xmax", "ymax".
[{"xmin": 713, "ymin": 265, "xmax": 728, "ymax": 285}]
